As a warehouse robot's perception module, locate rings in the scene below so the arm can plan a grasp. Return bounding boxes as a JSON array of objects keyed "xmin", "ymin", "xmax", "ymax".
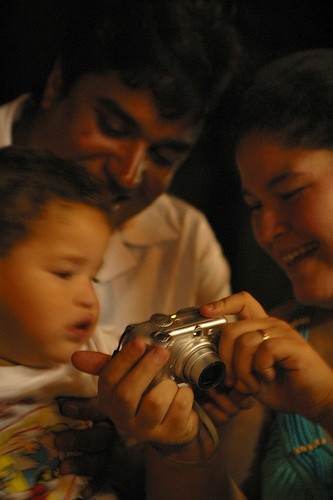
[{"xmin": 254, "ymin": 329, "xmax": 270, "ymax": 342}]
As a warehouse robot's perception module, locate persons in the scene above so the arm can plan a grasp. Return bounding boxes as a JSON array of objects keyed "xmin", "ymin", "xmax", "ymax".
[
  {"xmin": 67, "ymin": 46, "xmax": 333, "ymax": 500},
  {"xmin": 1, "ymin": 0, "xmax": 238, "ymax": 383},
  {"xmin": 0, "ymin": 144, "xmax": 258, "ymax": 500}
]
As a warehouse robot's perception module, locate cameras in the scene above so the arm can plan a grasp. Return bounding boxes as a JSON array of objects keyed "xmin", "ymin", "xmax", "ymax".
[{"xmin": 121, "ymin": 306, "xmax": 227, "ymax": 392}]
